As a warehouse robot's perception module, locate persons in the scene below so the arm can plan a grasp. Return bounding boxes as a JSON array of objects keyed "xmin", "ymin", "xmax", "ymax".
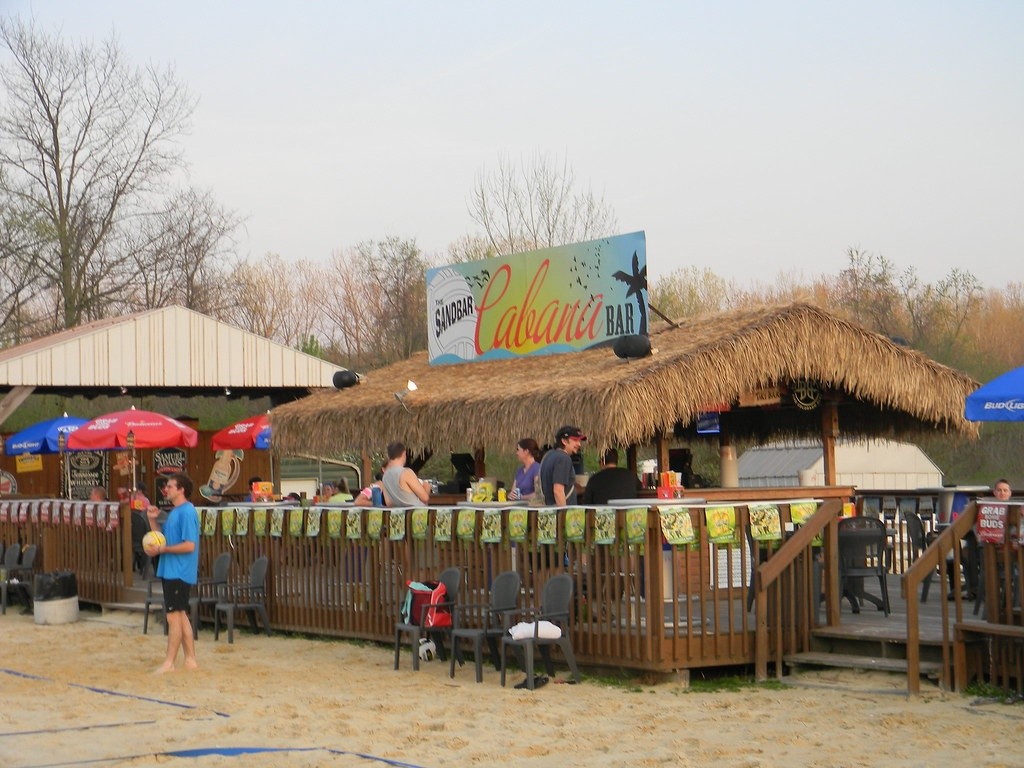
[
  {"xmin": 148, "ymin": 474, "xmax": 199, "ymax": 673},
  {"xmin": 356, "ymin": 460, "xmax": 387, "ymax": 506},
  {"xmin": 507, "ymin": 438, "xmax": 541, "ymax": 504},
  {"xmin": 127, "ymin": 482, "xmax": 150, "ymax": 510},
  {"xmin": 540, "ymin": 426, "xmax": 597, "ymax": 621},
  {"xmin": 383, "ymin": 441, "xmax": 431, "ymax": 508},
  {"xmin": 245, "ymin": 477, "xmax": 261, "ymax": 502},
  {"xmin": 89, "ymin": 486, "xmax": 108, "ymax": 501},
  {"xmin": 585, "ymin": 448, "xmax": 643, "ymax": 619},
  {"xmin": 962, "ymin": 480, "xmax": 1020, "ymax": 609}
]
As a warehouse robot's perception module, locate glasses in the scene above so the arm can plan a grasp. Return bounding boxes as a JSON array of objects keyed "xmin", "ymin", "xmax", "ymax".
[{"xmin": 516, "ymin": 447, "xmax": 520, "ymax": 451}]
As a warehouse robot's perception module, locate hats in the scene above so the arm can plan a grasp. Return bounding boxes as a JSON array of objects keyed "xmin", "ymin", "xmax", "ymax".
[{"xmin": 555, "ymin": 426, "xmax": 587, "ymax": 441}]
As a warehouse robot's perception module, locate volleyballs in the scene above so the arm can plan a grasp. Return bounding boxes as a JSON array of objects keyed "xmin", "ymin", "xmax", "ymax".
[
  {"xmin": 418, "ymin": 638, "xmax": 436, "ymax": 662},
  {"xmin": 142, "ymin": 531, "xmax": 166, "ymax": 556}
]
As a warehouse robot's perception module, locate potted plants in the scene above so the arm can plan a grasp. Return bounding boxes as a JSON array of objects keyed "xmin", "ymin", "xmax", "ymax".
[{"xmin": 32, "ymin": 569, "xmax": 79, "ymax": 624}]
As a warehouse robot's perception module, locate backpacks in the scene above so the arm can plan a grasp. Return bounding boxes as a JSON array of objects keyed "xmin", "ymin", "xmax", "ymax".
[{"xmin": 405, "ymin": 579, "xmax": 452, "ymax": 628}]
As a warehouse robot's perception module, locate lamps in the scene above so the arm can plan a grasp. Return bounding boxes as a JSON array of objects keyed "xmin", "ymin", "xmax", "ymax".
[
  {"xmin": 225, "ymin": 387, "xmax": 231, "ymax": 395},
  {"xmin": 121, "ymin": 386, "xmax": 128, "ymax": 393},
  {"xmin": 395, "ymin": 379, "xmax": 417, "ymax": 415}
]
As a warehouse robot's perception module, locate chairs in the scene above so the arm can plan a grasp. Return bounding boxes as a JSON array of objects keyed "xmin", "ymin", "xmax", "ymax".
[
  {"xmin": 0, "ymin": 543, "xmax": 37, "ymax": 615},
  {"xmin": 741, "ymin": 510, "xmax": 1019, "ymax": 620},
  {"xmin": 142, "ymin": 551, "xmax": 272, "ymax": 643},
  {"xmin": 131, "ymin": 511, "xmax": 151, "ymax": 572},
  {"xmin": 500, "ymin": 574, "xmax": 581, "ymax": 689},
  {"xmin": 394, "ymin": 566, "xmax": 465, "ymax": 672},
  {"xmin": 449, "ymin": 571, "xmax": 527, "ymax": 683}
]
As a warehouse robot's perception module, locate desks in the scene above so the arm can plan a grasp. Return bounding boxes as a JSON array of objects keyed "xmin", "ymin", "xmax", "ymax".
[
  {"xmin": 227, "ymin": 501, "xmax": 300, "ymax": 507},
  {"xmin": 429, "ymin": 493, "xmax": 467, "ymax": 504},
  {"xmin": 316, "ymin": 501, "xmax": 354, "ymax": 507},
  {"xmin": 608, "ymin": 497, "xmax": 706, "ymax": 506},
  {"xmin": 459, "ymin": 500, "xmax": 527, "ymax": 508}
]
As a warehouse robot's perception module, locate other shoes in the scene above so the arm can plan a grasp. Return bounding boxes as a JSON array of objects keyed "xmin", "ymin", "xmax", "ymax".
[{"xmin": 515, "ymin": 674, "xmax": 548, "ymax": 689}]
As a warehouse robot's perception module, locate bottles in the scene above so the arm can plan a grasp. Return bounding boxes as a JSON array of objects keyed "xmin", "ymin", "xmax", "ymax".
[
  {"xmin": 432, "ymin": 476, "xmax": 439, "ymax": 496},
  {"xmin": 372, "ymin": 484, "xmax": 381, "ymax": 507},
  {"xmin": 405, "ymin": 580, "xmax": 432, "ymax": 591},
  {"xmin": 207, "ymin": 448, "xmax": 235, "ymax": 495}
]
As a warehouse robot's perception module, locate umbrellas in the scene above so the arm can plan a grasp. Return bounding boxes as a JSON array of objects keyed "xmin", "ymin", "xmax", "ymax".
[
  {"xmin": 67, "ymin": 406, "xmax": 197, "ymax": 495},
  {"xmin": 965, "ymin": 365, "xmax": 1024, "ymax": 422},
  {"xmin": 210, "ymin": 413, "xmax": 274, "ymax": 485},
  {"xmin": 4, "ymin": 413, "xmax": 90, "ymax": 501}
]
